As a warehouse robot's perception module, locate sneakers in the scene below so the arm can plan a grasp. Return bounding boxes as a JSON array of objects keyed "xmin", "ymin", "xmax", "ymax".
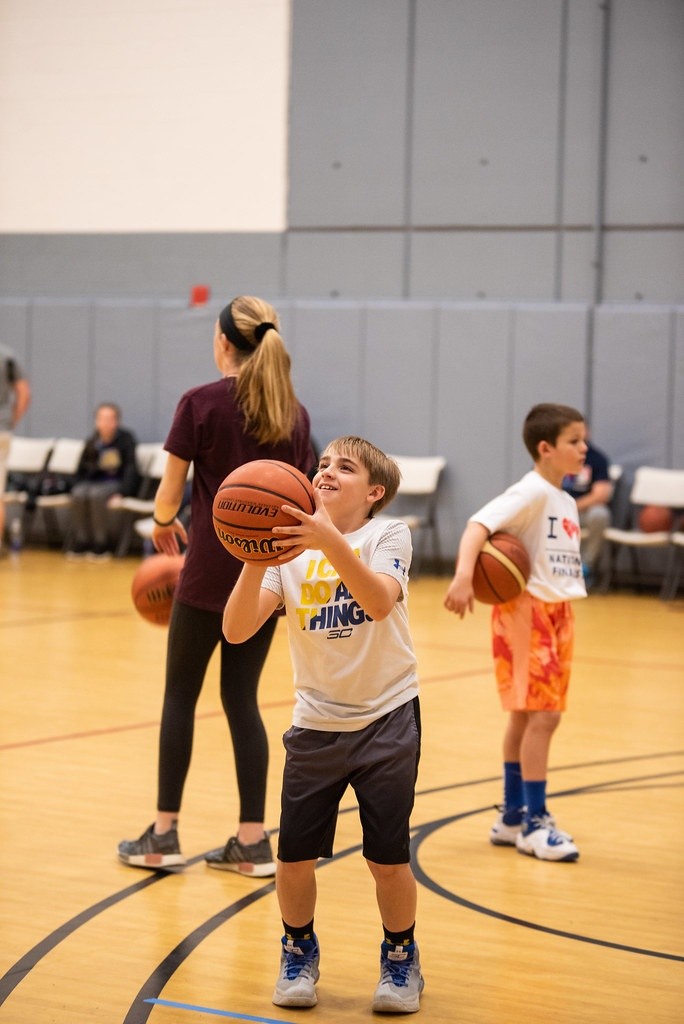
[
  {"xmin": 490, "ymin": 805, "xmax": 523, "ymax": 847},
  {"xmin": 272, "ymin": 931, "xmax": 321, "ymax": 1007},
  {"xmin": 117, "ymin": 819, "xmax": 187, "ymax": 867},
  {"xmin": 204, "ymin": 832, "xmax": 277, "ymax": 877},
  {"xmin": 516, "ymin": 812, "xmax": 579, "ymax": 862},
  {"xmin": 372, "ymin": 940, "xmax": 425, "ymax": 1013}
]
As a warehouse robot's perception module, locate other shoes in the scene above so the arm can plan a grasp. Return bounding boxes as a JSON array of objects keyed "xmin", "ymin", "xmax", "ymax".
[
  {"xmin": 68, "ymin": 544, "xmax": 89, "ymax": 555},
  {"xmin": 87, "ymin": 544, "xmax": 111, "ymax": 558}
]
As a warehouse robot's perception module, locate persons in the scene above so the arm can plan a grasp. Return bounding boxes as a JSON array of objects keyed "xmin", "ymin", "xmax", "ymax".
[
  {"xmin": 443, "ymin": 403, "xmax": 588, "ymax": 862},
  {"xmin": 561, "ymin": 442, "xmax": 614, "ymax": 589},
  {"xmin": 116, "ymin": 296, "xmax": 315, "ymax": 878},
  {"xmin": 221, "ymin": 437, "xmax": 424, "ymax": 1014},
  {"xmin": 67, "ymin": 404, "xmax": 140, "ymax": 561},
  {"xmin": 0, "ymin": 346, "xmax": 29, "ymax": 553}
]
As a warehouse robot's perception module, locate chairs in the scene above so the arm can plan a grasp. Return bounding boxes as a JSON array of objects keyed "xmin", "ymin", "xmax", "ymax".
[
  {"xmin": 375, "ymin": 455, "xmax": 446, "ymax": 578},
  {"xmin": 9, "ymin": 437, "xmax": 195, "ymax": 547},
  {"xmin": 581, "ymin": 466, "xmax": 684, "ymax": 599}
]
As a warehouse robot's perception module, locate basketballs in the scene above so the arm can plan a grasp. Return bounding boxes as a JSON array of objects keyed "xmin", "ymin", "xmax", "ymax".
[
  {"xmin": 130, "ymin": 552, "xmax": 194, "ymax": 627},
  {"xmin": 464, "ymin": 531, "xmax": 532, "ymax": 605},
  {"xmin": 638, "ymin": 503, "xmax": 672, "ymax": 532},
  {"xmin": 211, "ymin": 458, "xmax": 317, "ymax": 568}
]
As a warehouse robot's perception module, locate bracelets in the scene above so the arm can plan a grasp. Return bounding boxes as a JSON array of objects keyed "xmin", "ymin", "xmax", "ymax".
[{"xmin": 153, "ymin": 517, "xmax": 176, "ymax": 526}]
